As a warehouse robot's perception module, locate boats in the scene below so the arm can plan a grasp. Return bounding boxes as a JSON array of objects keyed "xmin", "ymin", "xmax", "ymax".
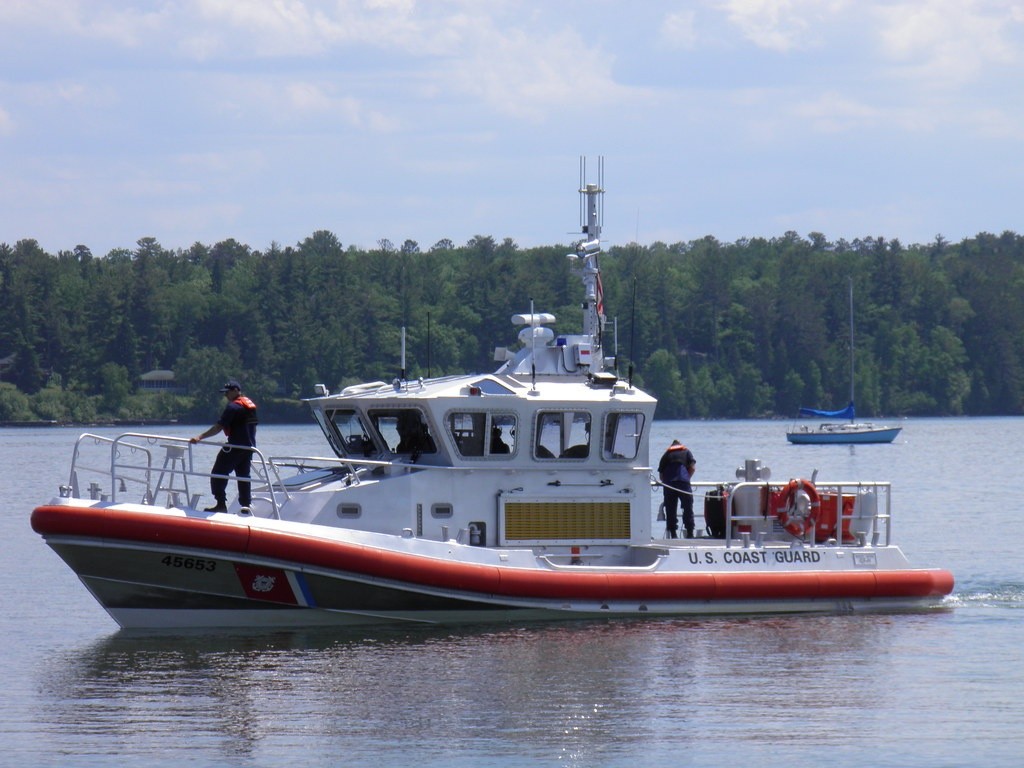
[{"xmin": 30, "ymin": 157, "xmax": 955, "ymax": 630}]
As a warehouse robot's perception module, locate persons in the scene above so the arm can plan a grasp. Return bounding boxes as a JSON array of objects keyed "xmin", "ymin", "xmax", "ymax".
[
  {"xmin": 389, "ymin": 411, "xmax": 612, "ymax": 459},
  {"xmin": 657, "ymin": 440, "xmax": 696, "ymax": 539},
  {"xmin": 188, "ymin": 381, "xmax": 257, "ymax": 514}
]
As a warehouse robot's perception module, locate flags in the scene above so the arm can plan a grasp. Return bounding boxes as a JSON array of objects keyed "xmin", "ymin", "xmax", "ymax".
[{"xmin": 597, "ymin": 274, "xmax": 607, "ymax": 319}]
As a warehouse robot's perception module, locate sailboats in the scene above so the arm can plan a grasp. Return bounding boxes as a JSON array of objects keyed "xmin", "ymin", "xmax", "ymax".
[{"xmin": 786, "ymin": 279, "xmax": 903, "ymax": 444}]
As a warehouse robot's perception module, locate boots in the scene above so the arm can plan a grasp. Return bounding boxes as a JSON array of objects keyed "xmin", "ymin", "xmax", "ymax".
[
  {"xmin": 204, "ymin": 499, "xmax": 227, "ymax": 513},
  {"xmin": 686, "ymin": 529, "xmax": 694, "ymax": 538},
  {"xmin": 671, "ymin": 529, "xmax": 678, "ymax": 539},
  {"xmin": 240, "ymin": 504, "xmax": 250, "ymax": 514}
]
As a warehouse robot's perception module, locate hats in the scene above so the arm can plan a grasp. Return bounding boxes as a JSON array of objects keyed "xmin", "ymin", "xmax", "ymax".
[{"xmin": 219, "ymin": 380, "xmax": 241, "ymax": 392}]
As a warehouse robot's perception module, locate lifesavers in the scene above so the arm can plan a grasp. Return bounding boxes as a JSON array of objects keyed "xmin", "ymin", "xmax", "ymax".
[{"xmin": 776, "ymin": 477, "xmax": 822, "ymax": 538}]
